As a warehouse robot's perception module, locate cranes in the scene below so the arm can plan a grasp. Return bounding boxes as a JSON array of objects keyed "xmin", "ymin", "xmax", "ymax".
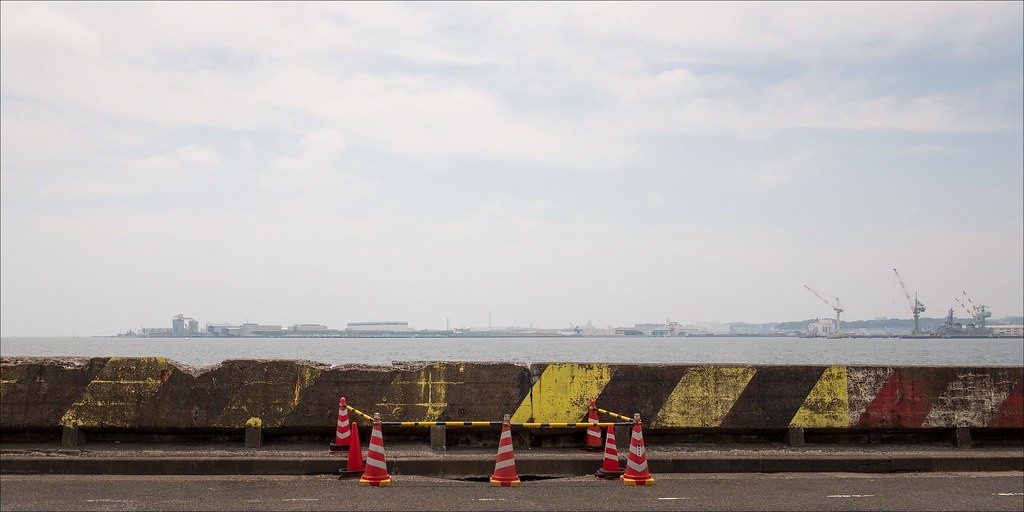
[
  {"xmin": 954, "ymin": 290, "xmax": 992, "ymax": 336},
  {"xmin": 803, "ymin": 284, "xmax": 843, "ymax": 333},
  {"xmin": 893, "ymin": 268, "xmax": 927, "ymax": 334}
]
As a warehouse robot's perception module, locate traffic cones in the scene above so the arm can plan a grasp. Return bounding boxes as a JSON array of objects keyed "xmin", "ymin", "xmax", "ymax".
[
  {"xmin": 337, "ymin": 422, "xmax": 366, "ymax": 478},
  {"xmin": 594, "ymin": 424, "xmax": 626, "ymax": 480},
  {"xmin": 358, "ymin": 413, "xmax": 393, "ymax": 487},
  {"xmin": 328, "ymin": 396, "xmax": 352, "ymax": 453},
  {"xmin": 619, "ymin": 412, "xmax": 639, "ymax": 486},
  {"xmin": 489, "ymin": 413, "xmax": 522, "ymax": 487},
  {"xmin": 580, "ymin": 399, "xmax": 605, "ymax": 452}
]
